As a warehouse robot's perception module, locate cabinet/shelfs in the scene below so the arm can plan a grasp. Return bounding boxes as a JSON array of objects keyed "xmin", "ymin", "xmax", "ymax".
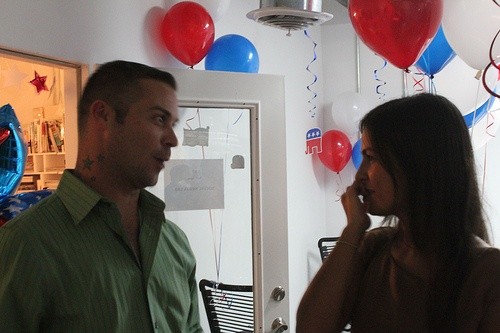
[{"xmin": 16, "ymin": 152, "xmax": 66, "ymax": 193}]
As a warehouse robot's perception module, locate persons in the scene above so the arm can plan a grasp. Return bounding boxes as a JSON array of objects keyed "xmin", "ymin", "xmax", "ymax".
[
  {"xmin": 0, "ymin": 59, "xmax": 205, "ymax": 333},
  {"xmin": 296, "ymin": 94, "xmax": 500, "ymax": 333}
]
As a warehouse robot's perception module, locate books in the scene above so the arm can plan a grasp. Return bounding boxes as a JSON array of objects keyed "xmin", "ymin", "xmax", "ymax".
[
  {"xmin": 21, "ymin": 155, "xmax": 34, "ymax": 173},
  {"xmin": 15, "ymin": 175, "xmax": 40, "ymax": 195},
  {"xmin": 44, "ymin": 178, "xmax": 62, "ymax": 188},
  {"xmin": 21, "ymin": 118, "xmax": 65, "ymax": 153},
  {"xmin": 47, "ymin": 164, "xmax": 65, "ymax": 172}
]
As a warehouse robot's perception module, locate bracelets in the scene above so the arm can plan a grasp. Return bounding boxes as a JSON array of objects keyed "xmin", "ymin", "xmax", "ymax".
[{"xmin": 336, "ymin": 240, "xmax": 359, "ymax": 249}]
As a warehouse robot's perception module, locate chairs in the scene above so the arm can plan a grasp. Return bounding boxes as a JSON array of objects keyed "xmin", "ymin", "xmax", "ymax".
[{"xmin": 199, "ymin": 277, "xmax": 253, "ymax": 333}]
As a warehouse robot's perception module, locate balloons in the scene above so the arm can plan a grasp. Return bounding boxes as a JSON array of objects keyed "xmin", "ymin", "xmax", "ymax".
[
  {"xmin": 317, "ymin": 94, "xmax": 371, "ymax": 174},
  {"xmin": 205, "ymin": 33, "xmax": 260, "ymax": 73},
  {"xmin": 160, "ymin": 0, "xmax": 215, "ymax": 69},
  {"xmin": 348, "ymin": 0, "xmax": 500, "ymax": 129}
]
